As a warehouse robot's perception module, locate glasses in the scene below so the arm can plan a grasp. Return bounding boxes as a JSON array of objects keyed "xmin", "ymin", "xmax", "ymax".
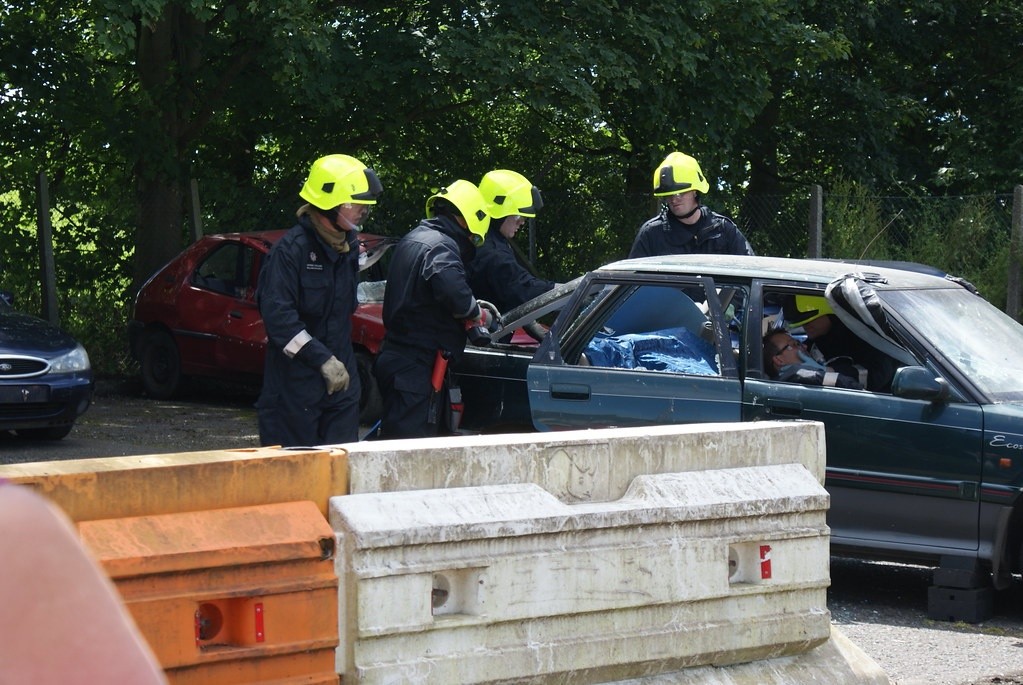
[
  {"xmin": 776, "ymin": 340, "xmax": 803, "ymax": 356},
  {"xmin": 673, "ymin": 193, "xmax": 691, "ymax": 198},
  {"xmin": 506, "ymin": 215, "xmax": 521, "ymax": 221}
]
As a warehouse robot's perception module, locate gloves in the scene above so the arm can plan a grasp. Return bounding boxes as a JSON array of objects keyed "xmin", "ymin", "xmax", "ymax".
[
  {"xmin": 554, "ymin": 283, "xmax": 565, "ymax": 288},
  {"xmin": 320, "ymin": 355, "xmax": 350, "ymax": 396},
  {"xmin": 484, "ymin": 309, "xmax": 492, "ymax": 328}
]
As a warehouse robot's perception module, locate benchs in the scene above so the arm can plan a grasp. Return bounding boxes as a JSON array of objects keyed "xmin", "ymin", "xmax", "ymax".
[{"xmin": 198, "ymin": 279, "xmax": 235, "ymax": 292}]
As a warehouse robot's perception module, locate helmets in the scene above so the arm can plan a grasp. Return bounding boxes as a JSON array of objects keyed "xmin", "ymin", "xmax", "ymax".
[
  {"xmin": 479, "ymin": 169, "xmax": 543, "ymax": 219},
  {"xmin": 426, "ymin": 179, "xmax": 491, "ymax": 246},
  {"xmin": 654, "ymin": 152, "xmax": 709, "ymax": 197},
  {"xmin": 298, "ymin": 153, "xmax": 377, "ymax": 211},
  {"xmin": 783, "ymin": 294, "xmax": 835, "ymax": 329}
]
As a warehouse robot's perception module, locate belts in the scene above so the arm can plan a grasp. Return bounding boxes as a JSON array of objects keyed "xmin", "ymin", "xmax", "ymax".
[{"xmin": 382, "ymin": 342, "xmax": 434, "ymax": 364}]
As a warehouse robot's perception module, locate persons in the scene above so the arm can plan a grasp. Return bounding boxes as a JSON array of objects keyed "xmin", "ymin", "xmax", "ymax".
[
  {"xmin": 780, "ymin": 294, "xmax": 894, "ymax": 391},
  {"xmin": 1, "ymin": 476, "xmax": 168, "ymax": 685},
  {"xmin": 370, "ymin": 178, "xmax": 498, "ymax": 441},
  {"xmin": 458, "ymin": 169, "xmax": 565, "ymax": 433},
  {"xmin": 254, "ymin": 153, "xmax": 383, "ymax": 449},
  {"xmin": 763, "ymin": 326, "xmax": 819, "ymax": 381},
  {"xmin": 629, "ymin": 152, "xmax": 755, "ymax": 326}
]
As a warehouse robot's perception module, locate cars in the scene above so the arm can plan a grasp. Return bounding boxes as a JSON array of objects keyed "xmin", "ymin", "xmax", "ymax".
[
  {"xmin": 444, "ymin": 253, "xmax": 1023, "ymax": 617},
  {"xmin": 129, "ymin": 229, "xmax": 553, "ymax": 438},
  {"xmin": 0, "ymin": 294, "xmax": 97, "ymax": 444}
]
